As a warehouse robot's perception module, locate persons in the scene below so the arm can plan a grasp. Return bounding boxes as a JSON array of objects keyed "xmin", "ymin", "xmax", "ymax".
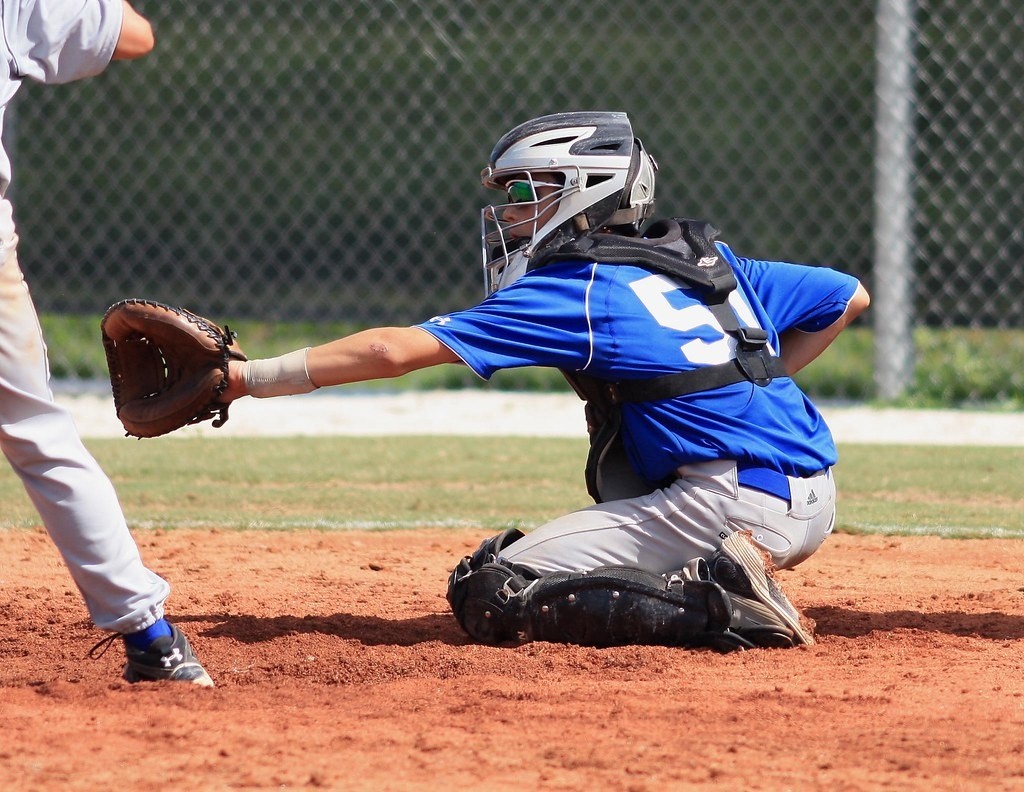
[
  {"xmin": 100, "ymin": 110, "xmax": 871, "ymax": 652},
  {"xmin": 0, "ymin": 0, "xmax": 216, "ymax": 687}
]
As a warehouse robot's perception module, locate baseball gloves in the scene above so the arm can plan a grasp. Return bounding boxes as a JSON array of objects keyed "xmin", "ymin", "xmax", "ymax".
[{"xmin": 99, "ymin": 298, "xmax": 241, "ymax": 439}]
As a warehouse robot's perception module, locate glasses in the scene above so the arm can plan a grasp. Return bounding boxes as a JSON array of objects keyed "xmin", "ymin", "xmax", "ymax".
[{"xmin": 504, "ymin": 178, "xmax": 568, "ymax": 205}]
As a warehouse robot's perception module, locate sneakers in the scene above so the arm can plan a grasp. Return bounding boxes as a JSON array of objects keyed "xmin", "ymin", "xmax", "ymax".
[
  {"xmin": 701, "ymin": 529, "xmax": 817, "ymax": 650},
  {"xmin": 89, "ymin": 623, "xmax": 215, "ymax": 688}
]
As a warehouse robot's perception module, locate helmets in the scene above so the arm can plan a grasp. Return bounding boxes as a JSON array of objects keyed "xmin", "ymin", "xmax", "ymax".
[{"xmin": 480, "ymin": 110, "xmax": 658, "ymax": 297}]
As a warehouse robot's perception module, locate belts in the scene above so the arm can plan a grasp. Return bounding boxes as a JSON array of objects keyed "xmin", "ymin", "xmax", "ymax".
[{"xmin": 736, "ymin": 462, "xmax": 830, "ymax": 501}]
